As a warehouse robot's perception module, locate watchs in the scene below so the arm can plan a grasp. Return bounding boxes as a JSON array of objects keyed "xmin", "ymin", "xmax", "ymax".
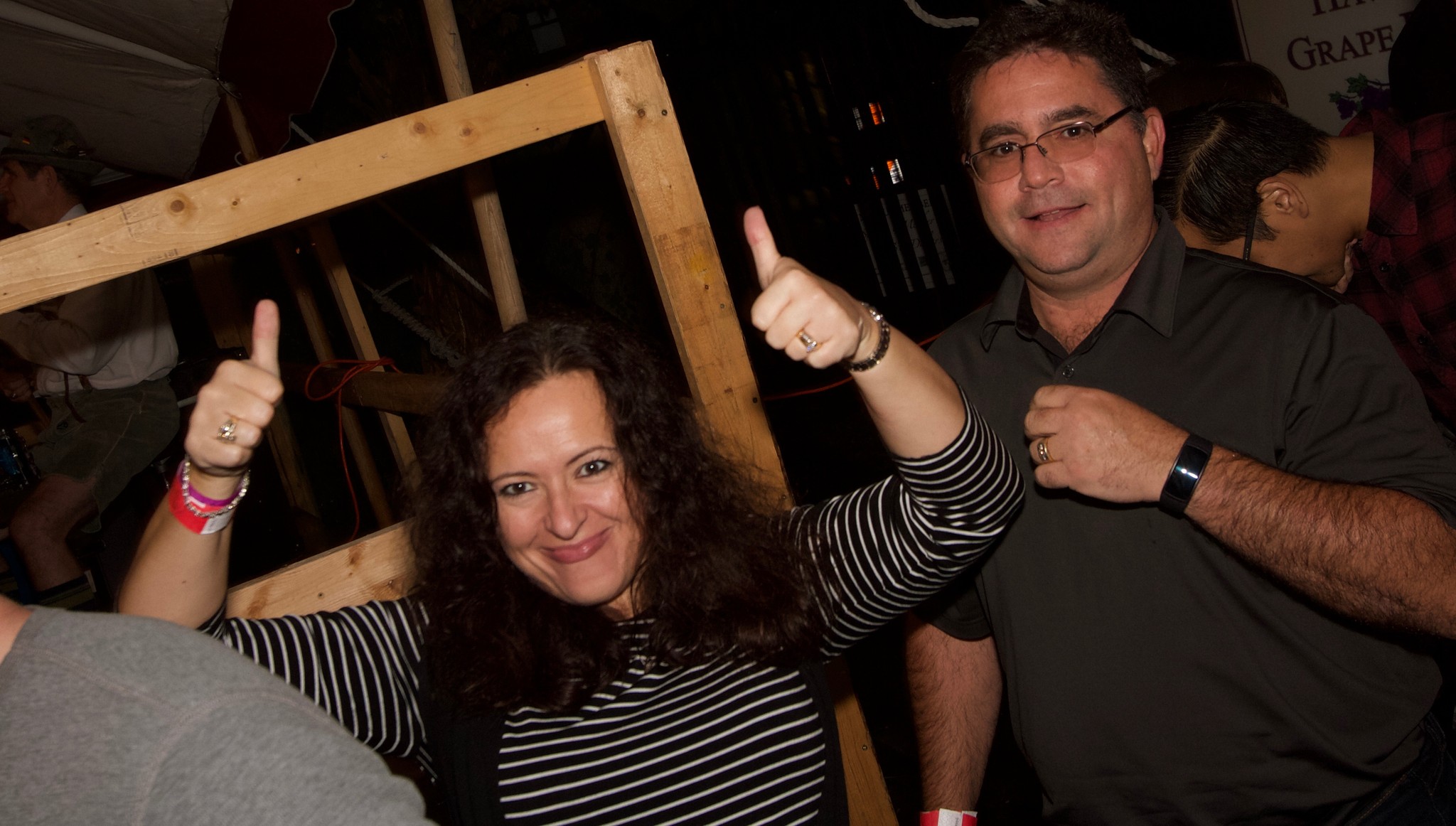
[{"xmin": 1158, "ymin": 433, "xmax": 1214, "ymax": 520}]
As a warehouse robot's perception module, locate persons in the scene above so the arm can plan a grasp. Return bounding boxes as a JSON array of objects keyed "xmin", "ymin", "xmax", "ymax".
[
  {"xmin": 0, "ymin": 591, "xmax": 434, "ymax": 826},
  {"xmin": 1151, "ymin": 99, "xmax": 1456, "ymax": 454},
  {"xmin": 119, "ymin": 205, "xmax": 1022, "ymax": 826},
  {"xmin": 0, "ymin": 113, "xmax": 181, "ymax": 611},
  {"xmin": 1386, "ymin": 0, "xmax": 1456, "ymax": 122},
  {"xmin": 904, "ymin": 2, "xmax": 1456, "ymax": 826},
  {"xmin": 1201, "ymin": 61, "xmax": 1289, "ymax": 110}
]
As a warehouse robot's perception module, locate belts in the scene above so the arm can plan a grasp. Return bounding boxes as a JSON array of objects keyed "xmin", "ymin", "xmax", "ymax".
[{"xmin": 1338, "ymin": 723, "xmax": 1433, "ymax": 826}]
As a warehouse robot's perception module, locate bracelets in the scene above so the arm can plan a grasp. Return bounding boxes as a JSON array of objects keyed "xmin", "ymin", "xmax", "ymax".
[
  {"xmin": 845, "ymin": 300, "xmax": 890, "ymax": 372},
  {"xmin": 167, "ymin": 455, "xmax": 251, "ymax": 535},
  {"xmin": 919, "ymin": 808, "xmax": 978, "ymax": 826}
]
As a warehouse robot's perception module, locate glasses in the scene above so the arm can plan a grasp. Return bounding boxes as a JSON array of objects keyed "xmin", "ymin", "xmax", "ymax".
[{"xmin": 964, "ymin": 103, "xmax": 1136, "ymax": 184}]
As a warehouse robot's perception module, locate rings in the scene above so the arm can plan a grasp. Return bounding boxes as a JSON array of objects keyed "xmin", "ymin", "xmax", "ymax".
[
  {"xmin": 1037, "ymin": 437, "xmax": 1054, "ymax": 464},
  {"xmin": 796, "ymin": 328, "xmax": 817, "ymax": 353},
  {"xmin": 13, "ymin": 394, "xmax": 16, "ymax": 398},
  {"xmin": 218, "ymin": 418, "xmax": 238, "ymax": 441}
]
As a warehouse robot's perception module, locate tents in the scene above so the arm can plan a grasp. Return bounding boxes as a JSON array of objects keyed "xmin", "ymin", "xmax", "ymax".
[{"xmin": 0, "ymin": 0, "xmax": 395, "ymax": 533}]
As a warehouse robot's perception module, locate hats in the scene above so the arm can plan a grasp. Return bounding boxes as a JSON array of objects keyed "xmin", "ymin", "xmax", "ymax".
[{"xmin": 0, "ymin": 113, "xmax": 105, "ymax": 175}]
